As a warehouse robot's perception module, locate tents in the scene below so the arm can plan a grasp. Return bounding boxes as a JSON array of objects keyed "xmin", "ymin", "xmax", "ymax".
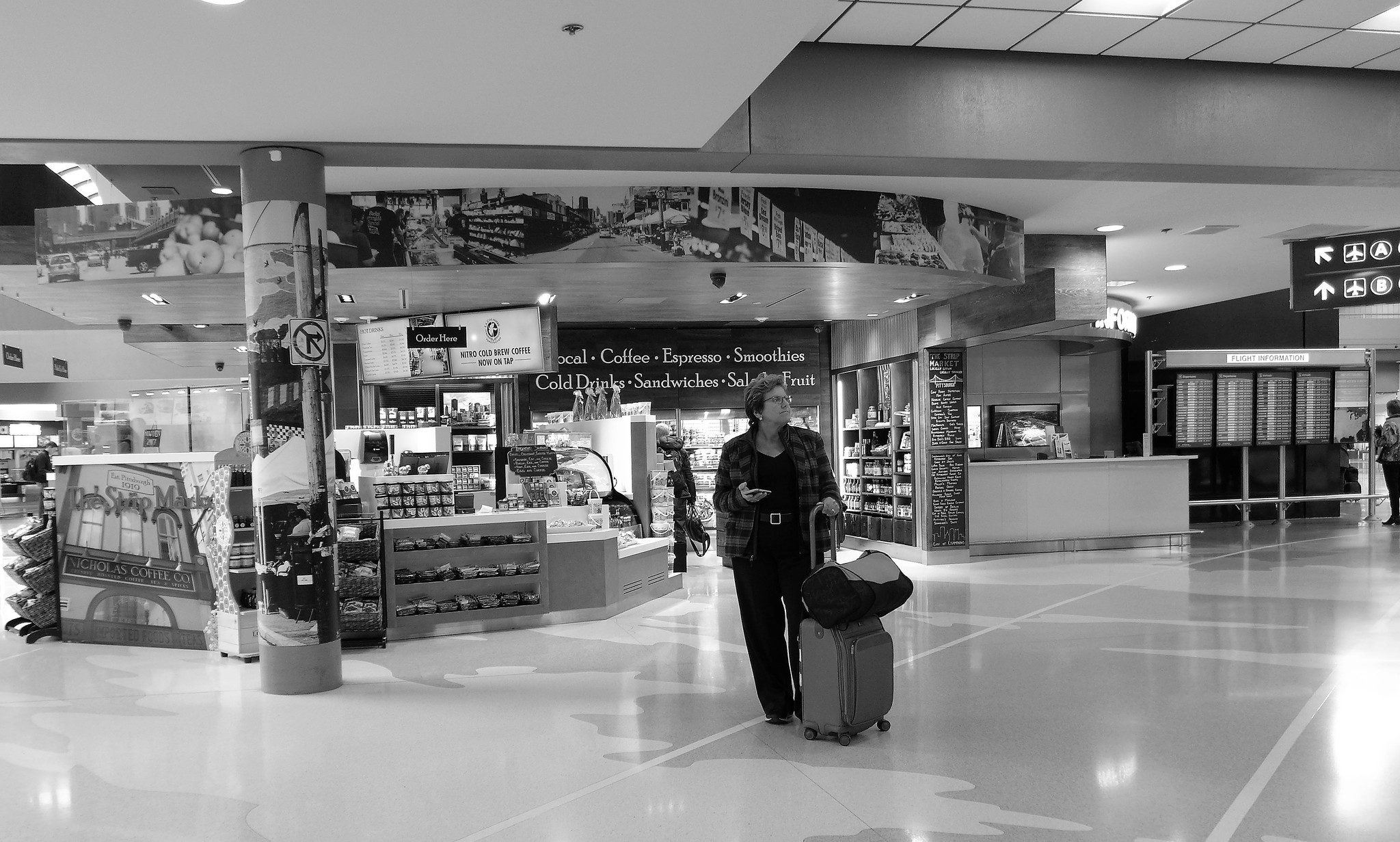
[{"xmin": 252, "ymin": 436, "xmax": 334, "ymax": 614}]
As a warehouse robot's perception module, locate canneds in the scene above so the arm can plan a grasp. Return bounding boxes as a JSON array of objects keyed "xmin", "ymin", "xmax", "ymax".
[
  {"xmin": 229, "ymin": 542, "xmax": 256, "ymax": 569},
  {"xmin": 498, "ymin": 493, "xmax": 525, "ymax": 512},
  {"xmin": 232, "ymin": 515, "xmax": 254, "ymax": 528},
  {"xmin": 241, "ymin": 588, "xmax": 257, "ymax": 609},
  {"xmin": 896, "ymin": 482, "xmax": 912, "ymax": 519},
  {"xmin": 867, "ymin": 406, "xmax": 877, "ymax": 420}
]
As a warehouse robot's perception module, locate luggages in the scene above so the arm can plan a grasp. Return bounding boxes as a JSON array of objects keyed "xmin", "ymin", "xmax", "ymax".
[{"xmin": 800, "ymin": 503, "xmax": 894, "ymax": 746}]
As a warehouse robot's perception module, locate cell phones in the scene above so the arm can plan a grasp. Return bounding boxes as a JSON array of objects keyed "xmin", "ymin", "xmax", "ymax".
[{"xmin": 742, "ymin": 488, "xmax": 772, "ymax": 497}]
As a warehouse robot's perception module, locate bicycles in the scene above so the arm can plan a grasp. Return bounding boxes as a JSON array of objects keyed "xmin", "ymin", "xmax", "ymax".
[{"xmin": 102, "ymin": 257, "xmax": 111, "ymax": 271}]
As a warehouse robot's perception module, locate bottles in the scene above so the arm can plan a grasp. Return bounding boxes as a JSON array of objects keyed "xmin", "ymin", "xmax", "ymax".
[
  {"xmin": 232, "ymin": 515, "xmax": 255, "ymax": 528},
  {"xmin": 242, "ymin": 587, "xmax": 257, "ymax": 609},
  {"xmin": 218, "ymin": 462, "xmax": 253, "ymax": 488},
  {"xmin": 608, "ymin": 504, "xmax": 636, "ymax": 529},
  {"xmin": 840, "ymin": 402, "xmax": 912, "ymax": 519}
]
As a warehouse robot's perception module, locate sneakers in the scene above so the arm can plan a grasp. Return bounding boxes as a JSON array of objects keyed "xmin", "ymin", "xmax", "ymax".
[{"xmin": 765, "ymin": 713, "xmax": 794, "ymax": 724}]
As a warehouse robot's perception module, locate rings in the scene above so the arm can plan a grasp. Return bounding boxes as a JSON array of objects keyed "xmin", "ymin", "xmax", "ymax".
[
  {"xmin": 755, "ymin": 499, "xmax": 757, "ymax": 501},
  {"xmin": 757, "ymin": 499, "xmax": 759, "ymax": 502},
  {"xmin": 831, "ymin": 509, "xmax": 836, "ymax": 513}
]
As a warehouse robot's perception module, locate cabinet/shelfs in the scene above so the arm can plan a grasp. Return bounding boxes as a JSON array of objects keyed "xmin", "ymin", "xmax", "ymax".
[
  {"xmin": 213, "ymin": 485, "xmax": 260, "ymax": 660},
  {"xmin": 338, "ymin": 515, "xmax": 385, "ymax": 649},
  {"xmin": 384, "ymin": 511, "xmax": 549, "ymax": 632},
  {"xmin": 836, "ymin": 359, "xmax": 921, "ymax": 546},
  {"xmin": 2, "ymin": 487, "xmax": 62, "ymax": 644},
  {"xmin": 689, "ymin": 438, "xmax": 732, "ymax": 530},
  {"xmin": 0, "ymin": 447, "xmax": 54, "ymax": 484}
]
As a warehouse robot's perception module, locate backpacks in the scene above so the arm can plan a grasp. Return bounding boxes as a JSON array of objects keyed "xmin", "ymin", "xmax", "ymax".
[{"xmin": 657, "ymin": 446, "xmax": 685, "ymax": 486}]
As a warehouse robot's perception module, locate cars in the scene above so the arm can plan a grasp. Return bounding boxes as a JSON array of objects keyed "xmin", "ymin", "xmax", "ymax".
[
  {"xmin": 36, "ymin": 259, "xmax": 42, "ymax": 277},
  {"xmin": 87, "ymin": 253, "xmax": 103, "ymax": 267},
  {"xmin": 75, "ymin": 245, "xmax": 140, "ymax": 262},
  {"xmin": 600, "ymin": 229, "xmax": 611, "ymax": 238}
]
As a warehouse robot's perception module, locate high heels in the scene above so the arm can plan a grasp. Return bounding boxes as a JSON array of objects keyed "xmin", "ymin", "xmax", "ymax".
[{"xmin": 1382, "ymin": 515, "xmax": 1400, "ymax": 525}]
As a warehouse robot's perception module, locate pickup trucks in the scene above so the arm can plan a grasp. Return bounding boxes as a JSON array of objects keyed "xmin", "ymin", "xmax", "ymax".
[{"xmin": 124, "ymin": 238, "xmax": 168, "ymax": 273}]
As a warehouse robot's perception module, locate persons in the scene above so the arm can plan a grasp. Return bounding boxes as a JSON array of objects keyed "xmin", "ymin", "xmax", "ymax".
[
  {"xmin": 263, "ymin": 509, "xmax": 311, "ymax": 560},
  {"xmin": 334, "ymin": 450, "xmax": 347, "ymax": 482},
  {"xmin": 1374, "ymin": 399, "xmax": 1400, "ymax": 526},
  {"xmin": 655, "ymin": 423, "xmax": 696, "ymax": 573},
  {"xmin": 34, "ymin": 442, "xmax": 57, "ymax": 519},
  {"xmin": 713, "ymin": 374, "xmax": 847, "ymax": 725}
]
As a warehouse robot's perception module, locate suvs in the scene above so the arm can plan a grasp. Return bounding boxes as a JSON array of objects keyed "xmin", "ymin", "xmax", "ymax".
[{"xmin": 46, "ymin": 252, "xmax": 80, "ymax": 283}]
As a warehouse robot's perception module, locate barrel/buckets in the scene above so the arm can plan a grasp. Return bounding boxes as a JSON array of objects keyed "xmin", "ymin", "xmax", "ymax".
[
  {"xmin": 587, "ymin": 489, "xmax": 603, "ymax": 531},
  {"xmin": 647, "ymin": 471, "xmax": 677, "ymax": 576}
]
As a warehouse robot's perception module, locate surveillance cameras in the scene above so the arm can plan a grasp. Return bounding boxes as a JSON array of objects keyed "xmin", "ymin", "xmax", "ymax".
[
  {"xmin": 215, "ymin": 362, "xmax": 225, "ymax": 371},
  {"xmin": 708, "ymin": 271, "xmax": 728, "ymax": 290},
  {"xmin": 117, "ymin": 319, "xmax": 133, "ymax": 332}
]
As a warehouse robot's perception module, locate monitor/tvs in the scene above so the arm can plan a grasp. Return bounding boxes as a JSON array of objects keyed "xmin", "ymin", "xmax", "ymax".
[
  {"xmin": 355, "ymin": 312, "xmax": 451, "ymax": 385},
  {"xmin": 966, "ymin": 405, "xmax": 982, "ymax": 449},
  {"xmin": 445, "ymin": 306, "xmax": 546, "ymax": 377},
  {"xmin": 398, "ymin": 452, "xmax": 450, "ymax": 476},
  {"xmin": 991, "ymin": 404, "xmax": 1061, "ymax": 448},
  {"xmin": 519, "ymin": 305, "xmax": 560, "ymax": 375}
]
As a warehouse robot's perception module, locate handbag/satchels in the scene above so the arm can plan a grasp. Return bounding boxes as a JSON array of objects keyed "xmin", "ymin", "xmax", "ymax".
[
  {"xmin": 685, "ymin": 504, "xmax": 711, "ymax": 556},
  {"xmin": 800, "ymin": 549, "xmax": 914, "ymax": 631}
]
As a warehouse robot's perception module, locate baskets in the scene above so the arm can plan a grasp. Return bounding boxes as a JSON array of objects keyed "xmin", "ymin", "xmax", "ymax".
[{"xmin": 567, "ymin": 490, "xmax": 588, "ymax": 506}]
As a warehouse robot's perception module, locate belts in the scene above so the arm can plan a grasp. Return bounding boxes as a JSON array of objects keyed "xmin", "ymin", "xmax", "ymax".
[{"xmin": 758, "ymin": 513, "xmax": 795, "ymax": 524}]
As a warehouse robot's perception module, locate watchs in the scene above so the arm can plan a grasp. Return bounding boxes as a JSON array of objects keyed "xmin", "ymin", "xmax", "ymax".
[{"xmin": 283, "ymin": 552, "xmax": 287, "ymax": 554}]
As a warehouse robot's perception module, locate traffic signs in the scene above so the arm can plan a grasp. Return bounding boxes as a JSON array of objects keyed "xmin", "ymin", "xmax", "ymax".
[{"xmin": 1289, "ymin": 227, "xmax": 1400, "ymax": 313}]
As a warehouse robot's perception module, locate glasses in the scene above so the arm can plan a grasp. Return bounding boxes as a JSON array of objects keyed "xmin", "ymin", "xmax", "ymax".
[
  {"xmin": 763, "ymin": 395, "xmax": 793, "ymax": 404},
  {"xmin": 1385, "ymin": 408, "xmax": 1387, "ymax": 410}
]
